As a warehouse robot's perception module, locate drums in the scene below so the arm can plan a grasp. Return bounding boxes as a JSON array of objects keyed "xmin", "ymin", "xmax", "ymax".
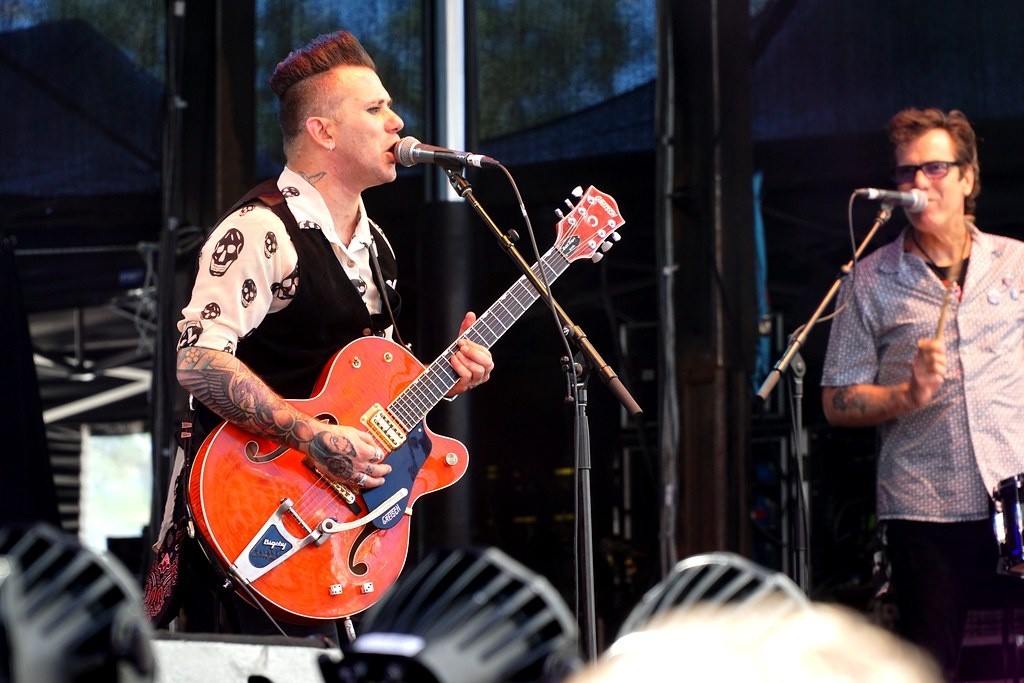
[{"xmin": 992, "ymin": 472, "xmax": 1022, "ymax": 574}]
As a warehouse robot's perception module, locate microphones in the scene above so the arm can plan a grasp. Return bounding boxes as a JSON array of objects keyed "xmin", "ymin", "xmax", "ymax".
[
  {"xmin": 394, "ymin": 136, "xmax": 499, "ymax": 169},
  {"xmin": 856, "ymin": 188, "xmax": 929, "ymax": 214}
]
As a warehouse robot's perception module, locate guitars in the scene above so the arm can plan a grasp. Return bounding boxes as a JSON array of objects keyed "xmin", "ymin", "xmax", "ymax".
[{"xmin": 188, "ymin": 185, "xmax": 627, "ymax": 621}]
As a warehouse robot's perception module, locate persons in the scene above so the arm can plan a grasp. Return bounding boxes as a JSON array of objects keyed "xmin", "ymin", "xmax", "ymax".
[
  {"xmin": 141, "ymin": 35, "xmax": 495, "ymax": 643},
  {"xmin": 821, "ymin": 108, "xmax": 1023, "ymax": 682}
]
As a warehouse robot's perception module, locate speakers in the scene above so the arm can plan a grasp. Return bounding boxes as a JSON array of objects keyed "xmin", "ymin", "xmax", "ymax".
[{"xmin": 119, "ymin": 628, "xmax": 343, "ymax": 683}]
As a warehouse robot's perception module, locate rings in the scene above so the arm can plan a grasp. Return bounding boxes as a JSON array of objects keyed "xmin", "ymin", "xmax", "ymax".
[
  {"xmin": 358, "ymin": 474, "xmax": 367, "ymax": 486},
  {"xmin": 370, "ymin": 450, "xmax": 379, "ymax": 462}
]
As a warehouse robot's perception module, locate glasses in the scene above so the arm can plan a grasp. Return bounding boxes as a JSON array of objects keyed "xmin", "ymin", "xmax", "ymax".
[{"xmin": 897, "ymin": 162, "xmax": 959, "ymax": 181}]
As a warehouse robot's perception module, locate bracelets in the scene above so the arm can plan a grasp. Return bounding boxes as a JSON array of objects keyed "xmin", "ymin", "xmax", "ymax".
[{"xmin": 443, "ymin": 394, "xmax": 459, "ymax": 402}]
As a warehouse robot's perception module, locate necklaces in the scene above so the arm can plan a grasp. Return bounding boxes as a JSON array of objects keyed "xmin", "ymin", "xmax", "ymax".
[{"xmin": 912, "ymin": 231, "xmax": 967, "ymax": 279}]
